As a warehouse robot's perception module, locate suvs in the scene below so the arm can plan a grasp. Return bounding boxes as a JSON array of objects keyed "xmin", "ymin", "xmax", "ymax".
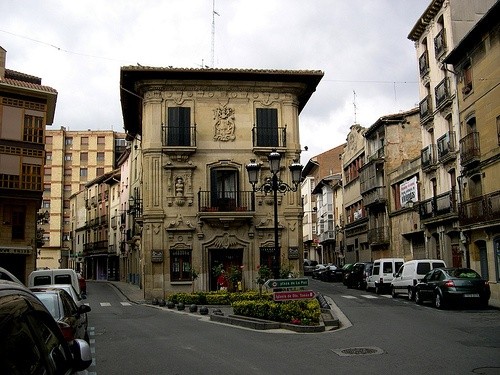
[{"xmin": 0, "ymin": 268, "xmax": 93, "ymax": 375}]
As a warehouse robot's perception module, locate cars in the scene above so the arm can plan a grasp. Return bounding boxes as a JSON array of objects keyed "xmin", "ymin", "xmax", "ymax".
[
  {"xmin": 76, "ymin": 273, "xmax": 87, "ymax": 295},
  {"xmin": 311, "ymin": 263, "xmax": 343, "ymax": 282},
  {"xmin": 412, "ymin": 268, "xmax": 491, "ymax": 310},
  {"xmin": 28, "ymin": 288, "xmax": 91, "ymax": 352}
]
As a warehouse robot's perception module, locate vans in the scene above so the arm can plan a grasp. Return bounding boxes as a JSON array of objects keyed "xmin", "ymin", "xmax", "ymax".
[
  {"xmin": 28, "ymin": 269, "xmax": 83, "ymax": 300},
  {"xmin": 304, "ymin": 260, "xmax": 318, "ymax": 274},
  {"xmin": 338, "ymin": 257, "xmax": 447, "ymax": 302}
]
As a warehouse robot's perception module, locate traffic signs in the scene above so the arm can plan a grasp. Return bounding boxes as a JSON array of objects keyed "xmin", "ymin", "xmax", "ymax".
[
  {"xmin": 273, "ymin": 290, "xmax": 317, "ymax": 301},
  {"xmin": 264, "ymin": 278, "xmax": 309, "ymax": 289}
]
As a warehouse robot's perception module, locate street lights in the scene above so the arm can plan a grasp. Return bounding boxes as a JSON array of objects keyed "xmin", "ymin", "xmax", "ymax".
[{"xmin": 244, "ymin": 148, "xmax": 305, "ymax": 304}]
